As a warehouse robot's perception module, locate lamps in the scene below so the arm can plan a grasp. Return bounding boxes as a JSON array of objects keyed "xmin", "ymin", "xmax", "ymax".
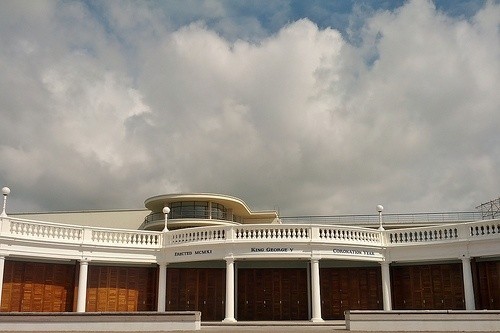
[
  {"xmin": 162, "ymin": 206, "xmax": 170, "ymax": 231},
  {"xmin": 376, "ymin": 204, "xmax": 386, "ymax": 230},
  {"xmin": 0, "ymin": 186, "xmax": 11, "ymax": 217}
]
